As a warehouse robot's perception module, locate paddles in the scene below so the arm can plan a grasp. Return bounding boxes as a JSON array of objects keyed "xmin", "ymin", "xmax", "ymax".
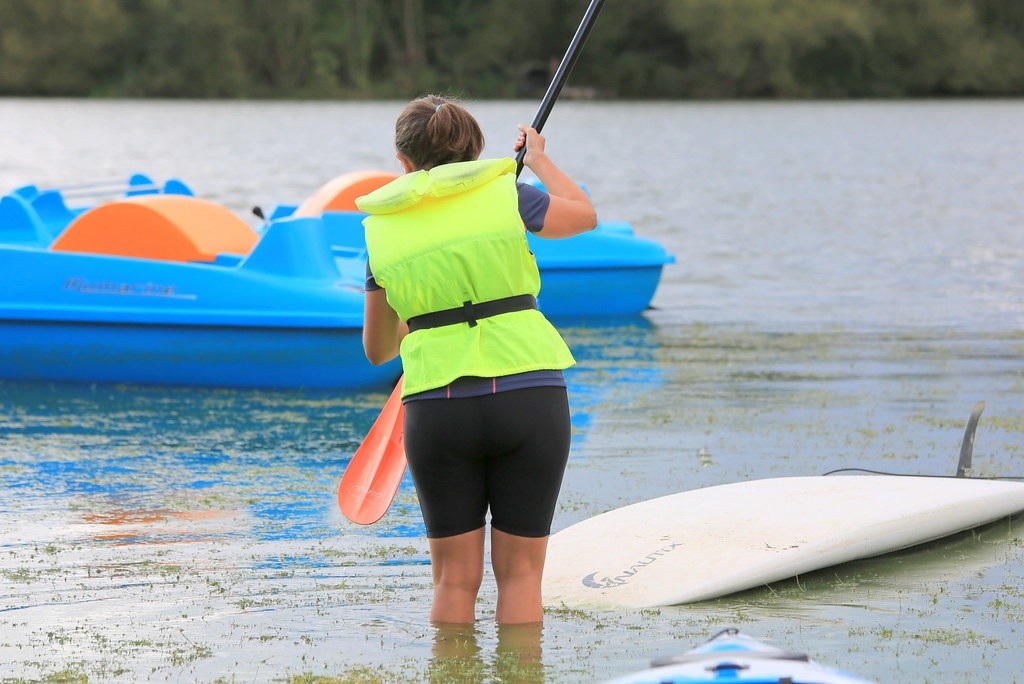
[{"xmin": 337, "ymin": 0, "xmax": 604, "ymax": 527}]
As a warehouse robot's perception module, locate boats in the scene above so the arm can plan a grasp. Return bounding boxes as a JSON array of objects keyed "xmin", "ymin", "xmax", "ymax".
[{"xmin": 0, "ymin": 167, "xmax": 681, "ymax": 388}]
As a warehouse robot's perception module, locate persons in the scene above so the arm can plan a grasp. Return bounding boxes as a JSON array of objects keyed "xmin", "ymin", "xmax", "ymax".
[{"xmin": 356, "ymin": 94, "xmax": 598, "ymax": 624}]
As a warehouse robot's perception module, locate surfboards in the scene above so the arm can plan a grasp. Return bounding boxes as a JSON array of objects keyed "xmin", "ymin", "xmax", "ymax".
[{"xmin": 538, "ymin": 400, "xmax": 1024, "ymax": 610}]
